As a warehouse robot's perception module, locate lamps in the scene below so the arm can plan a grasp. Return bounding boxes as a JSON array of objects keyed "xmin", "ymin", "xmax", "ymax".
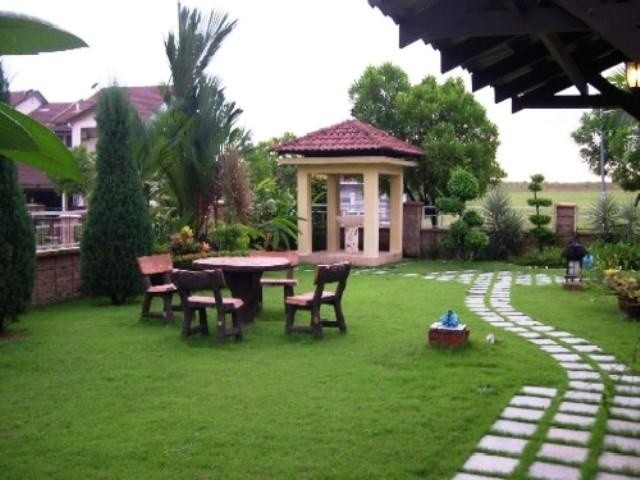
[{"xmin": 625, "ymin": 57, "xmax": 640, "ymax": 90}]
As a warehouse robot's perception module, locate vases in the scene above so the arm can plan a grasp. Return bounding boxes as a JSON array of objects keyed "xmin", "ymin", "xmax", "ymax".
[{"xmin": 618, "ymin": 297, "xmax": 640, "ymax": 317}]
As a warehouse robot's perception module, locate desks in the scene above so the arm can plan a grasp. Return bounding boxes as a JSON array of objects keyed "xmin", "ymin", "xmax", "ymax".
[{"xmin": 192, "ymin": 256, "xmax": 291, "ymax": 329}]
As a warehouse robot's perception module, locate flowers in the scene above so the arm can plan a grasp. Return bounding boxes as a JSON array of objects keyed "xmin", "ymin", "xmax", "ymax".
[{"xmin": 604, "ymin": 266, "xmax": 640, "ymax": 300}]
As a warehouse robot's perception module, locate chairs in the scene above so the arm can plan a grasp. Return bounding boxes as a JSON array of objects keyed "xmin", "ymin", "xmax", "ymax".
[
  {"xmin": 250, "ymin": 250, "xmax": 299, "ymax": 307},
  {"xmin": 171, "ymin": 267, "xmax": 244, "ymax": 343},
  {"xmin": 286, "ymin": 260, "xmax": 352, "ymax": 342},
  {"xmin": 136, "ymin": 253, "xmax": 184, "ymax": 323}
]
